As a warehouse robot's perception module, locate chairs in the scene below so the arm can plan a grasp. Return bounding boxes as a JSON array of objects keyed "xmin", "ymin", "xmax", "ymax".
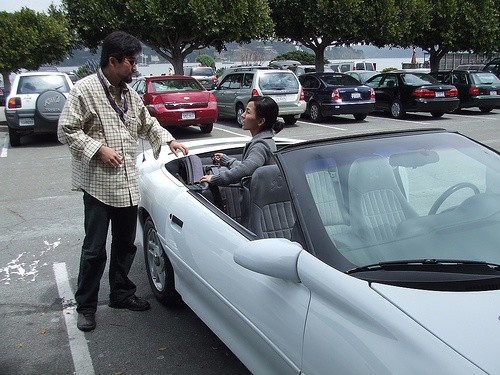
[
  {"xmin": 387, "ymin": 81, "xmax": 394, "ymax": 86},
  {"xmin": 305, "ymin": 168, "xmax": 351, "ymax": 235},
  {"xmin": 210, "ymin": 164, "xmax": 241, "ymax": 224},
  {"xmin": 348, "ymin": 157, "xmax": 420, "ymax": 227},
  {"xmin": 247, "ymin": 170, "xmax": 296, "ymax": 240}
]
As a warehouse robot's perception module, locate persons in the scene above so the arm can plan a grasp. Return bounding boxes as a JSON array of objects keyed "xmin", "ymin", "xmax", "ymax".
[
  {"xmin": 55, "ymin": 31, "xmax": 189, "ymax": 331},
  {"xmin": 168, "ymin": 67, "xmax": 173, "ymax": 75},
  {"xmin": 199, "ymin": 96, "xmax": 284, "ymax": 188}
]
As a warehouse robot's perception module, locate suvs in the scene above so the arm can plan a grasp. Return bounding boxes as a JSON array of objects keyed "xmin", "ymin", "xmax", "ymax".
[
  {"xmin": 269, "ymin": 61, "xmax": 334, "ymax": 77},
  {"xmin": 0, "ymin": 67, "xmax": 80, "ymax": 147},
  {"xmin": 209, "ymin": 66, "xmax": 307, "ymax": 127}
]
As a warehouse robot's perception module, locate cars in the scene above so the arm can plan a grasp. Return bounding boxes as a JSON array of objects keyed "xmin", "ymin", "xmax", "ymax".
[
  {"xmin": 162, "ymin": 66, "xmax": 217, "ymax": 90},
  {"xmin": 344, "ymin": 70, "xmax": 460, "ymax": 118},
  {"xmin": 455, "ymin": 58, "xmax": 500, "ymax": 70},
  {"xmin": 297, "ymin": 72, "xmax": 376, "ymax": 121},
  {"xmin": 128, "ymin": 76, "xmax": 218, "ymax": 133},
  {"xmin": 429, "ymin": 70, "xmax": 500, "ymax": 112}
]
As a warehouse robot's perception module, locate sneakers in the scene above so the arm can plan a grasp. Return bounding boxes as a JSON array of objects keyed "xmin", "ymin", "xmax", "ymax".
[
  {"xmin": 77, "ymin": 312, "xmax": 96, "ymax": 330},
  {"xmin": 108, "ymin": 296, "xmax": 150, "ymax": 311}
]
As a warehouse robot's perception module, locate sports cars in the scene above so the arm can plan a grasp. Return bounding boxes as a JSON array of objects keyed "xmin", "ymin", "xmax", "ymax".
[{"xmin": 136, "ymin": 126, "xmax": 500, "ymax": 375}]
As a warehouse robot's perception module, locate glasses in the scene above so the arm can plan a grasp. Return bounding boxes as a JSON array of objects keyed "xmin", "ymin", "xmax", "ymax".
[{"xmin": 124, "ymin": 59, "xmax": 137, "ymax": 65}]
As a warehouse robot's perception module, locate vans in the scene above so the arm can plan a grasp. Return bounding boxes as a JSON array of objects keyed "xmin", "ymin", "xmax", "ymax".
[{"xmin": 324, "ymin": 63, "xmax": 375, "ymax": 73}]
